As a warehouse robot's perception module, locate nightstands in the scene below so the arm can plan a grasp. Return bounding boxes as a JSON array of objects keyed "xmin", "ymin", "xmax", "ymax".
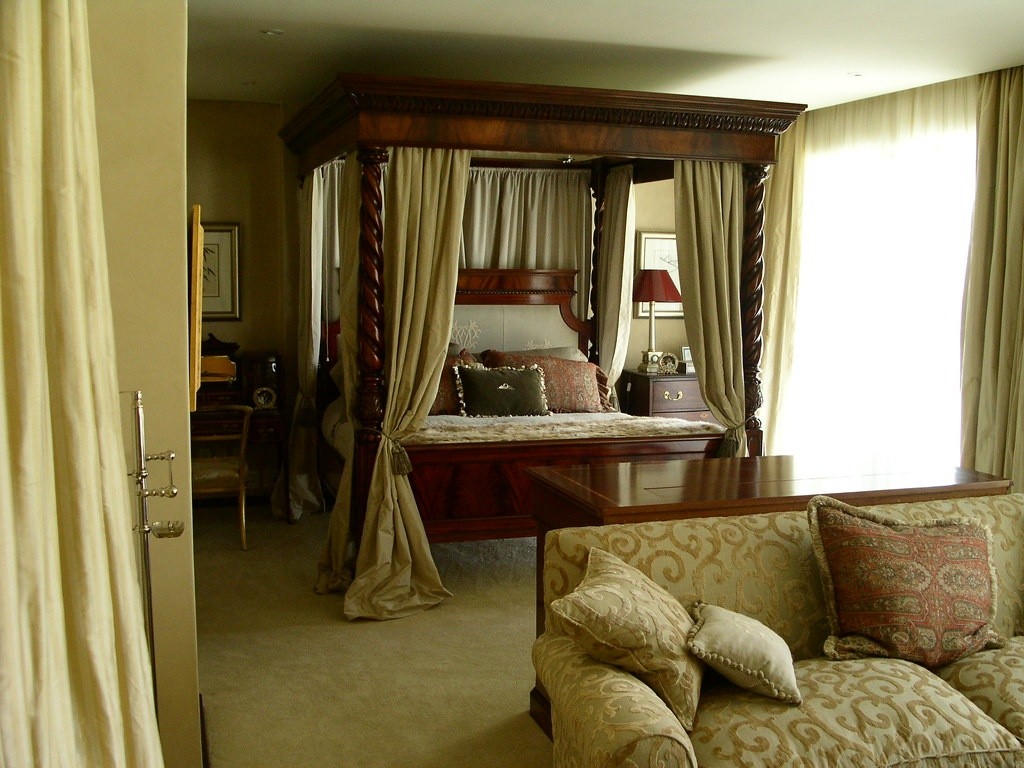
[
  {"xmin": 618, "ymin": 369, "xmax": 727, "ymax": 429},
  {"xmin": 190, "ymin": 411, "xmax": 289, "ymax": 521}
]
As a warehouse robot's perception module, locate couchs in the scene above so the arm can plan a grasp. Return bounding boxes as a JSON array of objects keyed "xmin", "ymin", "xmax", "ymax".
[{"xmin": 528, "ymin": 491, "xmax": 1024, "ymax": 767}]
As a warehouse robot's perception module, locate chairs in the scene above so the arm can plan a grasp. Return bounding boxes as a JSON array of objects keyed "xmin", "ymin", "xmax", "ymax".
[{"xmin": 191, "ymin": 406, "xmax": 253, "ymax": 552}]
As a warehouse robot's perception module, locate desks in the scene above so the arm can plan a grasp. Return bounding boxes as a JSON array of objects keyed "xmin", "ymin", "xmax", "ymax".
[{"xmin": 524, "ymin": 454, "xmax": 1013, "ymax": 746}]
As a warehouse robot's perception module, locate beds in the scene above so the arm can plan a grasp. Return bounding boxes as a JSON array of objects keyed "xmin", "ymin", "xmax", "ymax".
[{"xmin": 271, "ymin": 67, "xmax": 807, "ymax": 619}]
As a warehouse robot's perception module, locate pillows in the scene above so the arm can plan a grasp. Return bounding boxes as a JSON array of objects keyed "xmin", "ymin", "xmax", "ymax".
[
  {"xmin": 806, "ymin": 495, "xmax": 1006, "ymax": 670},
  {"xmin": 550, "ymin": 546, "xmax": 707, "ymax": 732},
  {"xmin": 330, "ymin": 341, "xmax": 613, "ymax": 417},
  {"xmin": 686, "ymin": 599, "xmax": 804, "ymax": 705}
]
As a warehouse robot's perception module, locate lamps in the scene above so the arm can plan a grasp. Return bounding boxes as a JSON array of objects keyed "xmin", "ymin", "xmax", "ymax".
[{"xmin": 632, "ymin": 269, "xmax": 682, "ymax": 372}]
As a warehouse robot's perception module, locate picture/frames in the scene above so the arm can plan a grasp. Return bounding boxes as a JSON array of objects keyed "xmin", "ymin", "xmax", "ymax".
[
  {"xmin": 634, "ymin": 231, "xmax": 685, "ymax": 319},
  {"xmin": 188, "ymin": 204, "xmax": 241, "ymax": 412}
]
